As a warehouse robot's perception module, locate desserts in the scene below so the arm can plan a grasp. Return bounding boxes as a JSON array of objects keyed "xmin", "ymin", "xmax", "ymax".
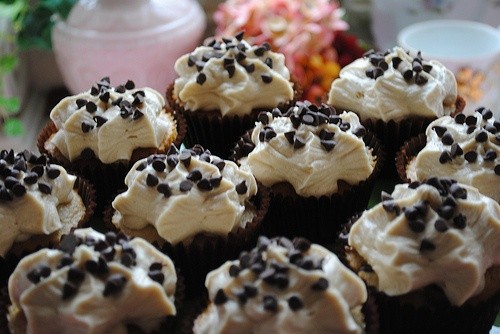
[{"xmin": 0, "ymin": 32, "xmax": 500, "ymax": 334}]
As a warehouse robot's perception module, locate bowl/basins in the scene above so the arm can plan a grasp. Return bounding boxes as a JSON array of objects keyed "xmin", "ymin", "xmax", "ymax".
[{"xmin": 396, "ymin": 17, "xmax": 500, "ymax": 76}]
